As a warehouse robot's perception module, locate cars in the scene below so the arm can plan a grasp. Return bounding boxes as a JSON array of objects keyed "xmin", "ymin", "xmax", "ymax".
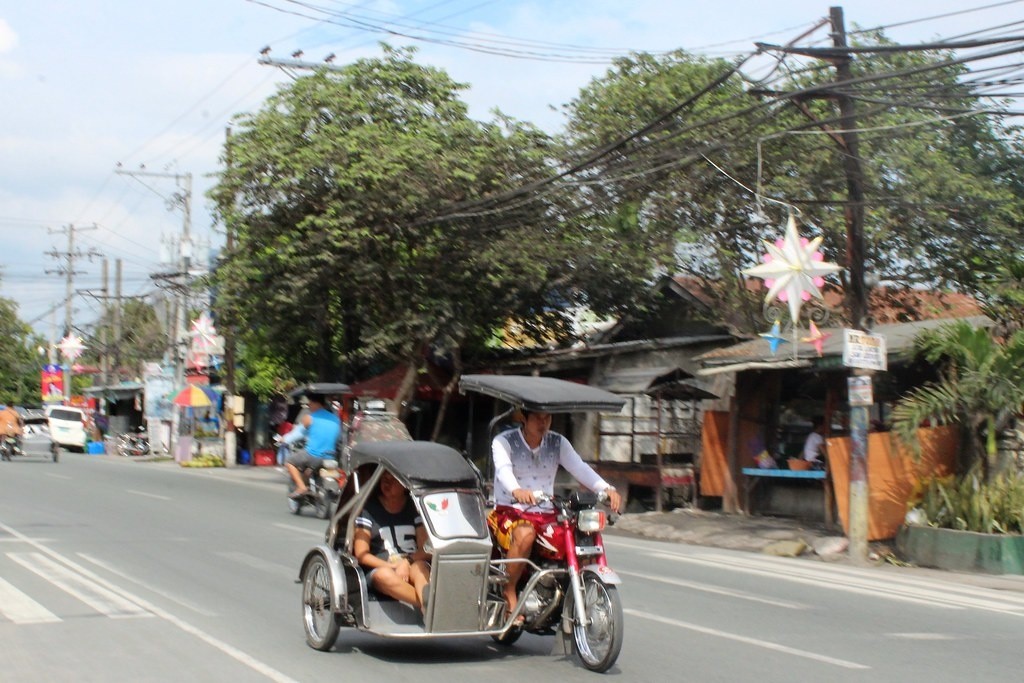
[{"xmin": 44, "ymin": 404, "xmax": 87, "ymax": 447}]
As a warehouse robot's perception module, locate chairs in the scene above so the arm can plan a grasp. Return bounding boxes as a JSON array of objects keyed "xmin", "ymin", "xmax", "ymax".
[{"xmin": 361, "ymin": 564, "xmax": 397, "ymax": 602}]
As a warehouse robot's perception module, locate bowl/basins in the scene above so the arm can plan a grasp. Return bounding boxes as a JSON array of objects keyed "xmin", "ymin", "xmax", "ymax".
[{"xmin": 787, "ymin": 459, "xmax": 812, "ymax": 470}]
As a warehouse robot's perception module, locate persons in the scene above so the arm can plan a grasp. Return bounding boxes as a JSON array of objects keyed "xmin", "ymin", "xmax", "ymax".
[
  {"xmin": 353, "ymin": 468, "xmax": 433, "ymax": 620},
  {"xmin": 278, "ymin": 394, "xmax": 343, "ymax": 498},
  {"xmin": 0, "ymin": 401, "xmax": 24, "ymax": 453},
  {"xmin": 488, "ymin": 405, "xmax": 622, "ymax": 627},
  {"xmin": 802, "ymin": 415, "xmax": 829, "ymax": 470}
]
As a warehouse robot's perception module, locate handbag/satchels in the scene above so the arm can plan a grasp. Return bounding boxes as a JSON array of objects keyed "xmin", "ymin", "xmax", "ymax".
[{"xmin": 18, "ymin": 417, "xmax": 24, "ymax": 427}]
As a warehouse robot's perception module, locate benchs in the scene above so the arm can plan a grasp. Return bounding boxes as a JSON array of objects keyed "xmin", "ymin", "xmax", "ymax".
[{"xmin": 741, "ymin": 468, "xmax": 834, "ymax": 523}]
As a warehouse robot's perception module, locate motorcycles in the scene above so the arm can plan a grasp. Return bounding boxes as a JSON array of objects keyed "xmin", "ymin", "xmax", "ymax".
[
  {"xmin": 274, "ymin": 440, "xmax": 347, "ymax": 520},
  {"xmin": 295, "ymin": 435, "xmax": 624, "ymax": 673}
]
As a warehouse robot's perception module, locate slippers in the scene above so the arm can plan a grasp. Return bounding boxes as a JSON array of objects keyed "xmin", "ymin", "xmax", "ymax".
[
  {"xmin": 503, "ymin": 594, "xmax": 525, "ymax": 626},
  {"xmin": 286, "ymin": 490, "xmax": 311, "ymax": 498}
]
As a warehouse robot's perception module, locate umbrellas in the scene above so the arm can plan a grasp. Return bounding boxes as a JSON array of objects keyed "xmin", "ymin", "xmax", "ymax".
[{"xmin": 167, "ymin": 384, "xmax": 221, "ymax": 408}]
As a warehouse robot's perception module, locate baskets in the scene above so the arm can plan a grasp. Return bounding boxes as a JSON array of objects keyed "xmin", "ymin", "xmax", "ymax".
[{"xmin": 788, "ymin": 451, "xmax": 812, "ymax": 471}]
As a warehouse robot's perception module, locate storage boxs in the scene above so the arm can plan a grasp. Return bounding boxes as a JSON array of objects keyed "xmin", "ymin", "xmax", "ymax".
[
  {"xmin": 242, "ymin": 450, "xmax": 250, "ymax": 464},
  {"xmin": 254, "ymin": 450, "xmax": 275, "ymax": 465},
  {"xmin": 87, "ymin": 441, "xmax": 104, "ymax": 454}
]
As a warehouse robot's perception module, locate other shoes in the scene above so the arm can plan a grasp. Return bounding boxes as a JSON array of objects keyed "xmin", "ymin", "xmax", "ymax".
[{"xmin": 422, "ymin": 583, "xmax": 429, "ymax": 607}]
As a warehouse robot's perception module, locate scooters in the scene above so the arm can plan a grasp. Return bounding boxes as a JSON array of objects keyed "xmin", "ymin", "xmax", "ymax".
[{"xmin": 0, "ymin": 435, "xmax": 19, "ymax": 461}]
